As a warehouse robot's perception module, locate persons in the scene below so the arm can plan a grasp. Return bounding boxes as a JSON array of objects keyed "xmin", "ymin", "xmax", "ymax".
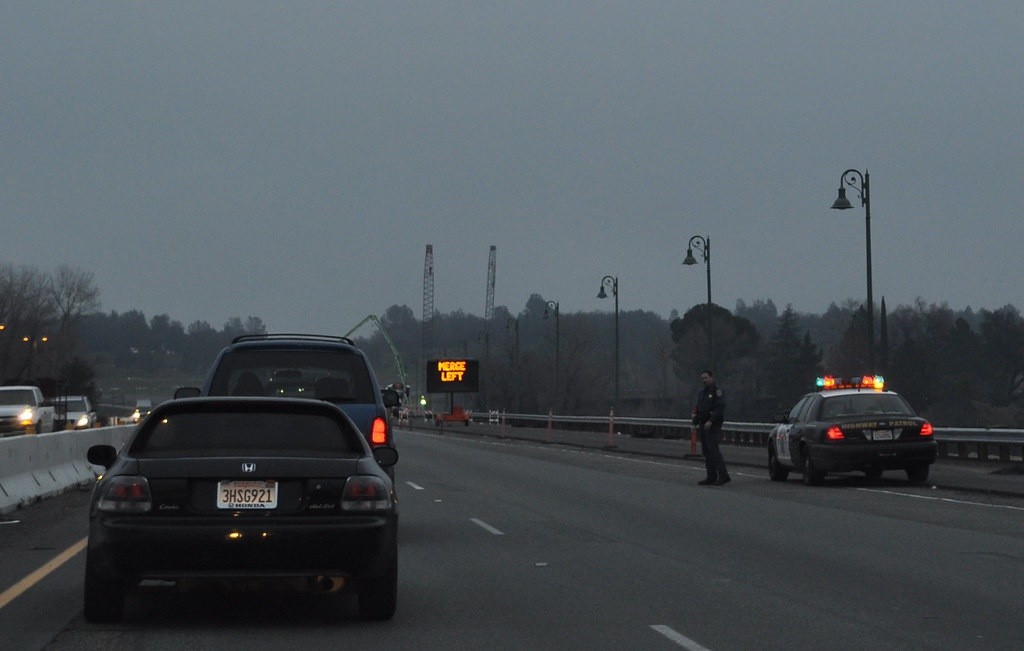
[{"xmin": 692, "ymin": 369, "xmax": 731, "ymax": 486}]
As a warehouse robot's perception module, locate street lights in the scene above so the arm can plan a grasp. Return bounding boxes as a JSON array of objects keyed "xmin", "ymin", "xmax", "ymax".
[
  {"xmin": 477, "ymin": 329, "xmax": 491, "ymax": 366},
  {"xmin": 597, "ymin": 275, "xmax": 620, "ymax": 417},
  {"xmin": 681, "ymin": 234, "xmax": 713, "ymax": 376},
  {"xmin": 542, "ymin": 300, "xmax": 560, "ymax": 415},
  {"xmin": 830, "ymin": 167, "xmax": 876, "ymax": 378},
  {"xmin": 506, "ymin": 316, "xmax": 520, "ymax": 362}
]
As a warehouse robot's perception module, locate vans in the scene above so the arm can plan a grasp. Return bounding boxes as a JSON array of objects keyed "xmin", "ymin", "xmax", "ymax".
[{"xmin": 200, "ymin": 333, "xmax": 396, "ymax": 498}]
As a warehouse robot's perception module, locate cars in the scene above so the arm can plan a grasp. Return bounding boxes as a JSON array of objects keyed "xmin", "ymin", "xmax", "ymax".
[
  {"xmin": 0, "ymin": 385, "xmax": 97, "ymax": 438},
  {"xmin": 82, "ymin": 395, "xmax": 399, "ymax": 624},
  {"xmin": 768, "ymin": 374, "xmax": 938, "ymax": 488}
]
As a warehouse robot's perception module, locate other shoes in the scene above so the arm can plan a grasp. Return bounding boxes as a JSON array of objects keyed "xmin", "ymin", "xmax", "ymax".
[
  {"xmin": 699, "ymin": 478, "xmax": 718, "ymax": 485},
  {"xmin": 718, "ymin": 477, "xmax": 730, "ymax": 485}
]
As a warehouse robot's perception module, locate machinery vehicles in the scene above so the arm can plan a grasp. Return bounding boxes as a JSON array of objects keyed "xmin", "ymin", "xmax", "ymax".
[{"xmin": 339, "ymin": 314, "xmax": 413, "ymax": 430}]
